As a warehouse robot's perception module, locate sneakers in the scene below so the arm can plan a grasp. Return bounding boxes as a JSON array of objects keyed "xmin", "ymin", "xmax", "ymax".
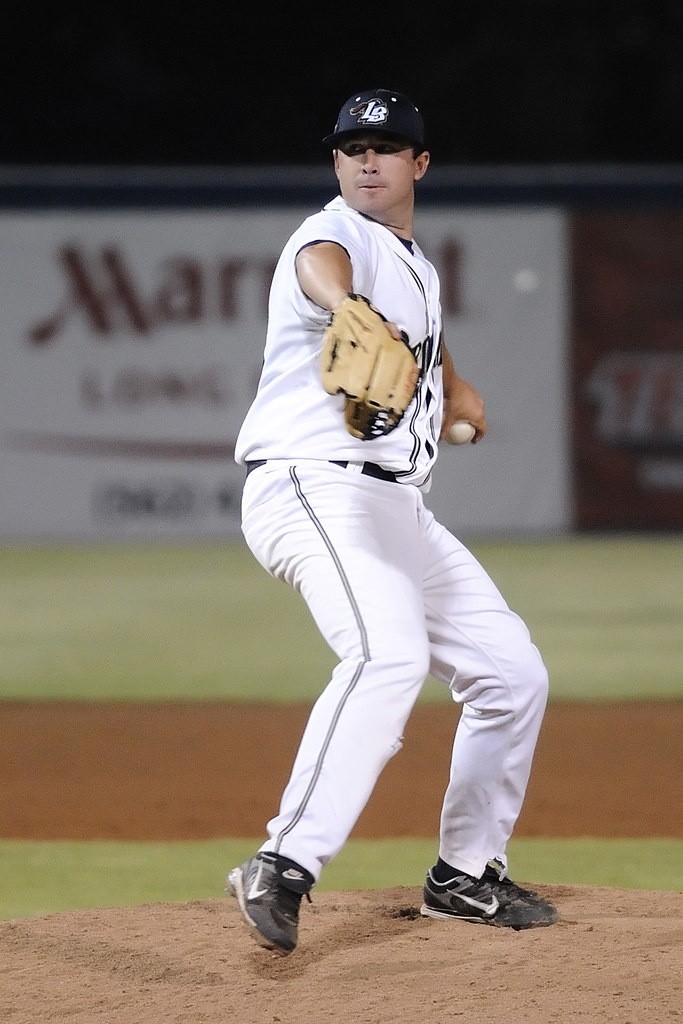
[
  {"xmin": 224, "ymin": 852, "xmax": 315, "ymax": 959},
  {"xmin": 420, "ymin": 863, "xmax": 559, "ymax": 931}
]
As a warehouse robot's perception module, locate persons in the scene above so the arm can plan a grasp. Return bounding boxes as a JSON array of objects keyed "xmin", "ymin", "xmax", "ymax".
[{"xmin": 223, "ymin": 89, "xmax": 563, "ymax": 959}]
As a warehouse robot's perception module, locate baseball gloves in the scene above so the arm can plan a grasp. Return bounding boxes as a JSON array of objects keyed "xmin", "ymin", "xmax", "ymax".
[{"xmin": 318, "ymin": 292, "xmax": 419, "ymax": 443}]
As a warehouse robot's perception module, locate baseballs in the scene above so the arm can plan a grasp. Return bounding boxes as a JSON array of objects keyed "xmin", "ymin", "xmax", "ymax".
[{"xmin": 446, "ymin": 421, "xmax": 476, "ymax": 445}]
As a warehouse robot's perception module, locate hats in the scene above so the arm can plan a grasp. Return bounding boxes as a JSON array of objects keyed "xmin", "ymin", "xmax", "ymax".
[{"xmin": 322, "ymin": 89, "xmax": 425, "ymax": 147}]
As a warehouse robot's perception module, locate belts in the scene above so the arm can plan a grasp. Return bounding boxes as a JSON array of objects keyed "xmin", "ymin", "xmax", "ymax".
[{"xmin": 245, "ymin": 460, "xmax": 401, "ymax": 484}]
